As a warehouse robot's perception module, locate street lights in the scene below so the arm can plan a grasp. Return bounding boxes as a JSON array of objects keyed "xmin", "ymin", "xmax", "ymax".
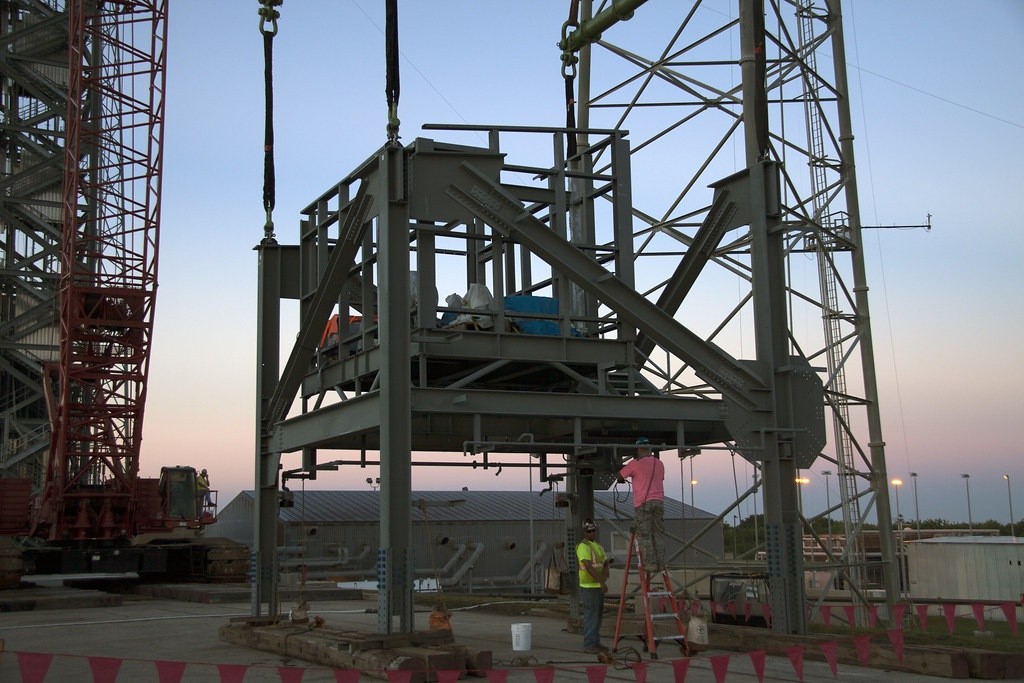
[
  {"xmin": 961, "ymin": 473, "xmax": 972, "ymax": 536},
  {"xmin": 892, "ymin": 480, "xmax": 902, "ymax": 515},
  {"xmin": 821, "ymin": 470, "xmax": 832, "ymax": 553},
  {"xmin": 1003, "ymin": 475, "xmax": 1014, "ymax": 536},
  {"xmin": 909, "ymin": 472, "xmax": 920, "ymax": 540},
  {"xmin": 797, "ymin": 478, "xmax": 810, "ymax": 514},
  {"xmin": 691, "ymin": 480, "xmax": 696, "ymax": 535}
]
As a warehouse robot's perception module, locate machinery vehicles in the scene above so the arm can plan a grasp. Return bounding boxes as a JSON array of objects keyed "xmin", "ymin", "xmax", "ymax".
[{"xmin": 0, "ymin": 0, "xmax": 252, "ymax": 612}]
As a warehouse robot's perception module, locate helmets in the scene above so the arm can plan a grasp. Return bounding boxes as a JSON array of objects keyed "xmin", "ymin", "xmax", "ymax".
[
  {"xmin": 582, "ymin": 517, "xmax": 597, "ymax": 530},
  {"xmin": 635, "ymin": 436, "xmax": 650, "ymax": 444}
]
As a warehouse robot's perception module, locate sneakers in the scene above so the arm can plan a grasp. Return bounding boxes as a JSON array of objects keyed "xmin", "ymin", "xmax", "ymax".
[{"xmin": 585, "ymin": 644, "xmax": 609, "ymax": 653}]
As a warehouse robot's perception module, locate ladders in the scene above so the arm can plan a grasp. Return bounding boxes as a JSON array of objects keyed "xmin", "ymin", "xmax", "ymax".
[{"xmin": 609, "ymin": 519, "xmax": 695, "ymax": 662}]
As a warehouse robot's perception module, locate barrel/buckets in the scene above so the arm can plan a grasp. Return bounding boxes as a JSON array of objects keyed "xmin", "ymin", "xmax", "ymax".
[{"xmin": 510, "ymin": 623, "xmax": 532, "ymax": 651}]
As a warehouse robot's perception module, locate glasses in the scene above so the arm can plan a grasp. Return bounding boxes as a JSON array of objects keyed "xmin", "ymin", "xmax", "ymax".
[{"xmin": 585, "ymin": 529, "xmax": 596, "ymax": 533}]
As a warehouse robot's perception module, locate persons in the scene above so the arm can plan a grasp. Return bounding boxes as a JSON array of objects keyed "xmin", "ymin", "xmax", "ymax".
[
  {"xmin": 574, "ymin": 518, "xmax": 612, "ymax": 654},
  {"xmin": 197, "ymin": 469, "xmax": 216, "ymax": 520},
  {"xmin": 617, "ymin": 436, "xmax": 667, "ymax": 573}
]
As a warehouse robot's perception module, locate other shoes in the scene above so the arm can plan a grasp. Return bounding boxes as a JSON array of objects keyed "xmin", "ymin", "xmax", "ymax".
[
  {"xmin": 208, "ymin": 502, "xmax": 216, "ymax": 506},
  {"xmin": 644, "ymin": 564, "xmax": 664, "ymax": 572}
]
